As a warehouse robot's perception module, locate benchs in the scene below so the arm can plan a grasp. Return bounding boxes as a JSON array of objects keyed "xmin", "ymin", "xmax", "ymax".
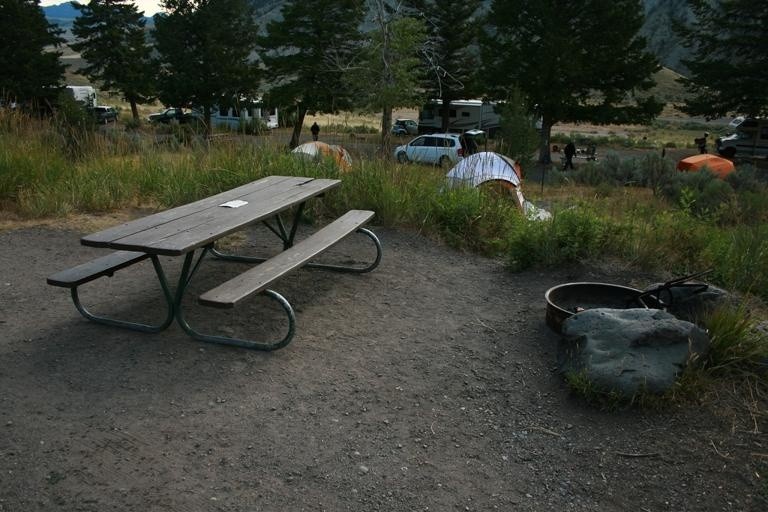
[
  {"xmin": 198, "ymin": 209, "xmax": 375, "ymax": 309},
  {"xmin": 46, "ymin": 250, "xmax": 149, "ymax": 288}
]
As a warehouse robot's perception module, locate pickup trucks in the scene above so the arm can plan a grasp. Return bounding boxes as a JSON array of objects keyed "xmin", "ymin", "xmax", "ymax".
[{"xmin": 147, "ymin": 106, "xmax": 205, "ymax": 126}]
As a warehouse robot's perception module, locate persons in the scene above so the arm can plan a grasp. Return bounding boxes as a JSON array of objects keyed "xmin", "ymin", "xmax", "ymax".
[
  {"xmin": 563, "ymin": 139, "xmax": 575, "ymax": 171},
  {"xmin": 695, "ymin": 133, "xmax": 710, "ymax": 157},
  {"xmin": 310, "ymin": 121, "xmax": 321, "ymax": 143}
]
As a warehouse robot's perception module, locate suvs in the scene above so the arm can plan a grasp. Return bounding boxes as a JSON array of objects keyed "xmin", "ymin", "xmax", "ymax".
[{"xmin": 395, "ymin": 129, "xmax": 487, "ymax": 169}]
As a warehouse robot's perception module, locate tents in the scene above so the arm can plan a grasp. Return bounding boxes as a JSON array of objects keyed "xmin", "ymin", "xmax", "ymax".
[
  {"xmin": 289, "ymin": 143, "xmax": 351, "ymax": 174},
  {"xmin": 437, "ymin": 150, "xmax": 525, "ymax": 215},
  {"xmin": 676, "ymin": 154, "xmax": 735, "ymax": 182}
]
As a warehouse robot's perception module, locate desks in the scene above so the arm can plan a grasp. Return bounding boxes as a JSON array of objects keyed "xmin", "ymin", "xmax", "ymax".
[{"xmin": 71, "ymin": 174, "xmax": 382, "ymax": 351}]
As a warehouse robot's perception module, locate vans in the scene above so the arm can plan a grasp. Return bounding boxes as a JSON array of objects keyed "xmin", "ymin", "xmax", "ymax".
[
  {"xmin": 392, "ymin": 118, "xmax": 419, "ymax": 136},
  {"xmin": 87, "ymin": 105, "xmax": 118, "ymax": 123}
]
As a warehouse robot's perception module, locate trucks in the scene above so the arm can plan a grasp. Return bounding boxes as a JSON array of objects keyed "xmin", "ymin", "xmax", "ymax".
[{"xmin": 715, "ymin": 116, "xmax": 768, "ymax": 158}]
